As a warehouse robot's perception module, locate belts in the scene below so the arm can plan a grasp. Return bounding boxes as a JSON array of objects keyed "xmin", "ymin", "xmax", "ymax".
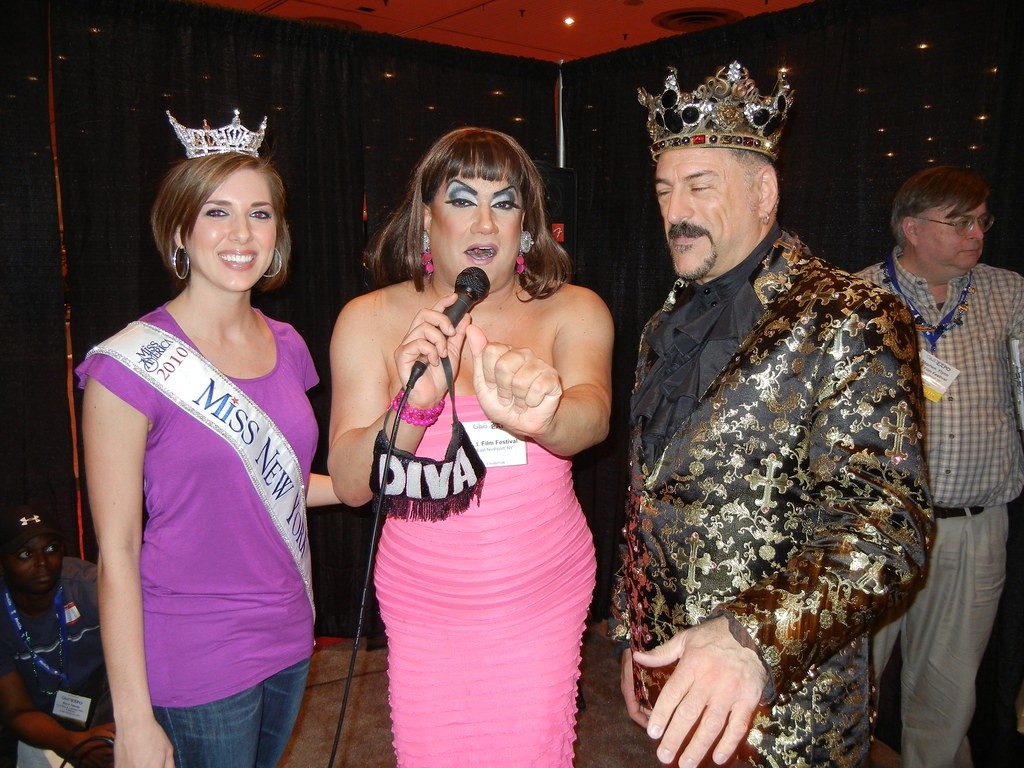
[{"xmin": 931, "ymin": 505, "xmax": 985, "ymax": 519}]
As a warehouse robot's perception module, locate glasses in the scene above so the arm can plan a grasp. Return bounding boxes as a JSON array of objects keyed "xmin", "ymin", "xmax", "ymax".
[{"xmin": 910, "ymin": 213, "xmax": 994, "ymax": 236}]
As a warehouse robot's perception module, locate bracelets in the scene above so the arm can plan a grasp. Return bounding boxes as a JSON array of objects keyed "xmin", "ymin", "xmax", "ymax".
[{"xmin": 393, "ymin": 387, "xmax": 445, "ymax": 427}]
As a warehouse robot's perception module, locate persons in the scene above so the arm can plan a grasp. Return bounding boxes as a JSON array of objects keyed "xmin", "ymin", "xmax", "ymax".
[
  {"xmin": 77, "ymin": 110, "xmax": 344, "ymax": 768},
  {"xmin": 851, "ymin": 165, "xmax": 1024, "ymax": 767},
  {"xmin": 326, "ymin": 125, "xmax": 614, "ymax": 766},
  {"xmin": 608, "ymin": 58, "xmax": 935, "ymax": 768},
  {"xmin": 0, "ymin": 504, "xmax": 116, "ymax": 768}
]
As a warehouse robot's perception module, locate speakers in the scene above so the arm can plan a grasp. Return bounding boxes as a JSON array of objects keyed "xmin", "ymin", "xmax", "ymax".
[{"xmin": 531, "ymin": 160, "xmax": 576, "ymax": 286}]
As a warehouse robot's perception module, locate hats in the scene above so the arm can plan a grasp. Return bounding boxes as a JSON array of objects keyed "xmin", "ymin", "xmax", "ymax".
[{"xmin": 0, "ymin": 504, "xmax": 72, "ymax": 553}]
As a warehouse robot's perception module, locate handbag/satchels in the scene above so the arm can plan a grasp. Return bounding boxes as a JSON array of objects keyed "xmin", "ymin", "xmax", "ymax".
[{"xmin": 370, "ymin": 357, "xmax": 486, "ymax": 523}]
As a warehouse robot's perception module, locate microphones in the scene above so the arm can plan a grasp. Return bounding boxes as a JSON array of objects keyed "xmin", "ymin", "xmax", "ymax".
[{"xmin": 408, "ymin": 266, "xmax": 491, "ymax": 388}]
